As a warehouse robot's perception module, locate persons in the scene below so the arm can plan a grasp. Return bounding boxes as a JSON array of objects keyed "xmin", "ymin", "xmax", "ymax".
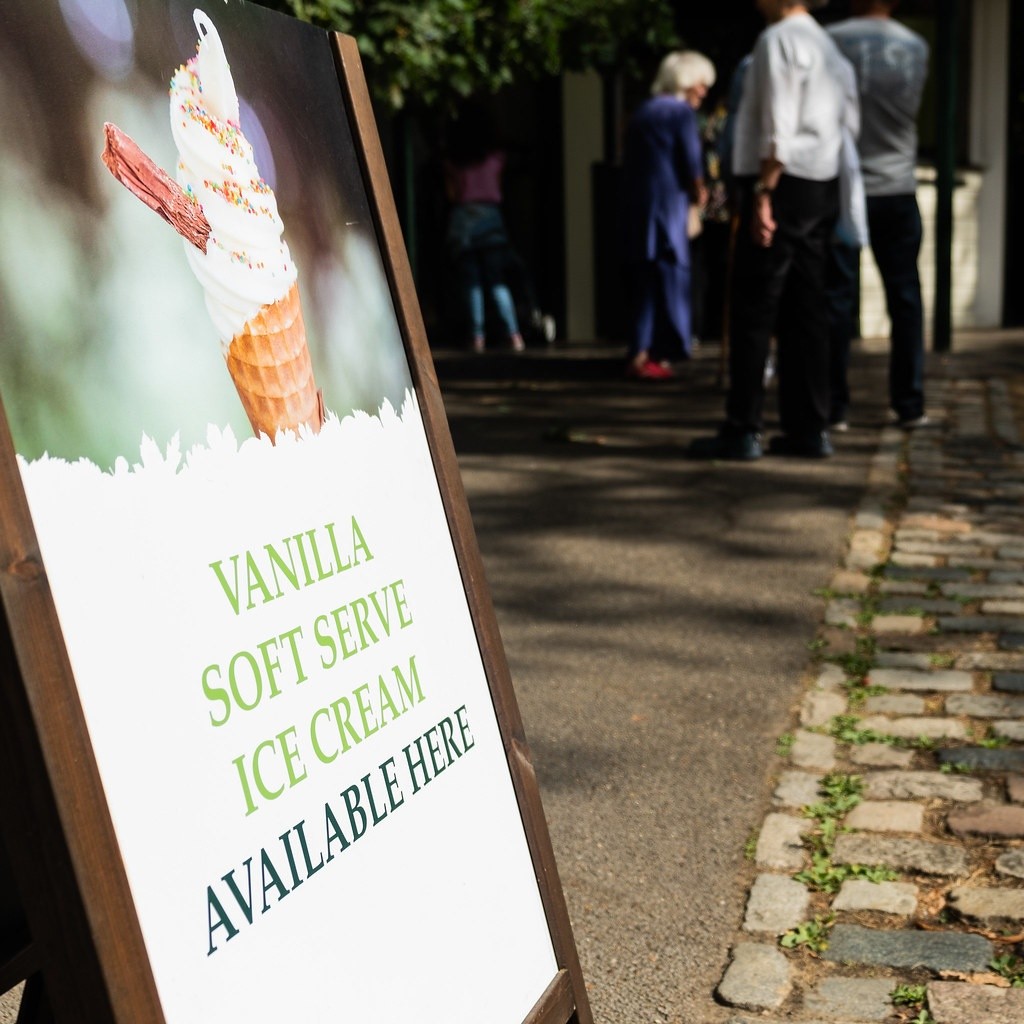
[
  {"xmin": 444, "ymin": 135, "xmax": 526, "ymax": 352},
  {"xmin": 685, "ymin": 0, "xmax": 858, "ymax": 461},
  {"xmin": 716, "ymin": 0, "xmax": 931, "ymax": 433},
  {"xmin": 623, "ymin": 52, "xmax": 715, "ymax": 380}
]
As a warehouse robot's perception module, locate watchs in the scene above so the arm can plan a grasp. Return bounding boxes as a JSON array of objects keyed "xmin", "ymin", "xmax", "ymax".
[{"xmin": 753, "ymin": 180, "xmax": 774, "ymax": 197}]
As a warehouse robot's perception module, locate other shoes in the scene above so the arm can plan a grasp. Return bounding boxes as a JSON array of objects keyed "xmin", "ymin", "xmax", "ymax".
[
  {"xmin": 691, "ymin": 427, "xmax": 761, "ymax": 459},
  {"xmin": 624, "ymin": 357, "xmax": 668, "ymax": 381},
  {"xmin": 771, "ymin": 431, "xmax": 833, "ymax": 457},
  {"xmin": 898, "ymin": 414, "xmax": 940, "ymax": 426},
  {"xmin": 830, "ymin": 420, "xmax": 850, "ymax": 432}
]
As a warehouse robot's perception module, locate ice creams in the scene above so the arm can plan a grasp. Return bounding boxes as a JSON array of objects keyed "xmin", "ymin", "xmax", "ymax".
[{"xmin": 103, "ymin": 10, "xmax": 324, "ymax": 441}]
